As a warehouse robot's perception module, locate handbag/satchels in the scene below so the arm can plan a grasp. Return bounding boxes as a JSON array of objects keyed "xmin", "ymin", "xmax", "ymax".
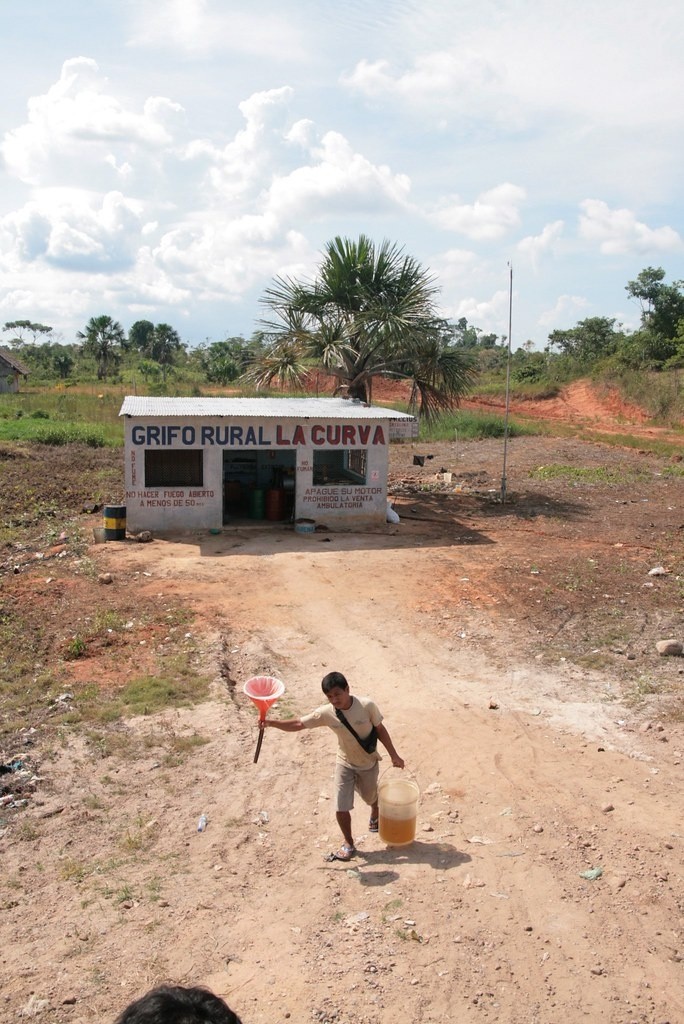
[{"xmin": 360, "ymin": 726, "xmax": 379, "ymax": 755}]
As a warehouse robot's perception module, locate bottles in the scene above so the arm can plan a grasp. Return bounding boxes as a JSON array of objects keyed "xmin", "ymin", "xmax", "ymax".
[{"xmin": 198, "ymin": 814, "xmax": 206, "ymax": 832}]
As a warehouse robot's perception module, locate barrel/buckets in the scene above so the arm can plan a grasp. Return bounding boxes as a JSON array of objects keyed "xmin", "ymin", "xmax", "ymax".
[
  {"xmin": 376, "ymin": 764, "xmax": 420, "ymax": 846},
  {"xmin": 103, "ymin": 505, "xmax": 126, "ymax": 540},
  {"xmin": 248, "ymin": 488, "xmax": 285, "ymax": 521}
]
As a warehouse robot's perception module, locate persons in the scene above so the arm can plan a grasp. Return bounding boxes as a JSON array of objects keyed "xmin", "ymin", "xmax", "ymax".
[
  {"xmin": 113, "ymin": 983, "xmax": 245, "ymax": 1024},
  {"xmin": 259, "ymin": 672, "xmax": 406, "ymax": 858}
]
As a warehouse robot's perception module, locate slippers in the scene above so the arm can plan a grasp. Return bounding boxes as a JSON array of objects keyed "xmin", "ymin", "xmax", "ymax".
[
  {"xmin": 335, "ymin": 844, "xmax": 356, "ymax": 860},
  {"xmin": 368, "ymin": 816, "xmax": 378, "ymax": 832}
]
